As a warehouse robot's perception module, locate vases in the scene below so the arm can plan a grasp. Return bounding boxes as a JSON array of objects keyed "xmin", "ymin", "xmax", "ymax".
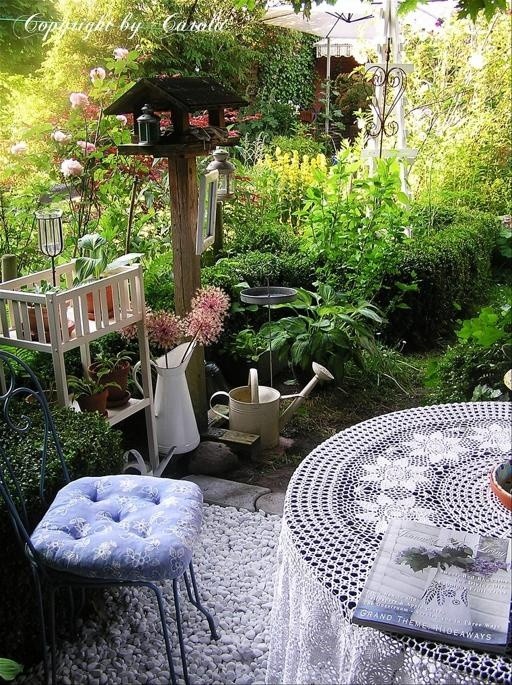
[
  {"xmin": 240, "ymin": 285, "xmax": 297, "ymax": 324},
  {"xmin": 150, "ymin": 340, "xmax": 203, "ymax": 454}
]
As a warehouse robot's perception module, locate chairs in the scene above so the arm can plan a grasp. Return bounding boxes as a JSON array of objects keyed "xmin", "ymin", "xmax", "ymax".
[{"xmin": 0, "ymin": 351, "xmax": 223, "ymax": 685}]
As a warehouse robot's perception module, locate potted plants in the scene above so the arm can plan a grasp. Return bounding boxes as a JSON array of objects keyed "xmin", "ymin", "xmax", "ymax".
[
  {"xmin": 15, "ymin": 277, "xmax": 75, "ymax": 343},
  {"xmin": 71, "ymin": 233, "xmax": 146, "ymax": 320},
  {"xmin": 65, "ymin": 366, "xmax": 122, "ymax": 417},
  {"xmin": 228, "ymin": 326, "xmax": 270, "ymax": 381},
  {"xmin": 258, "ymin": 280, "xmax": 387, "ymax": 392},
  {"xmin": 86, "ymin": 348, "xmax": 136, "ymax": 408}
]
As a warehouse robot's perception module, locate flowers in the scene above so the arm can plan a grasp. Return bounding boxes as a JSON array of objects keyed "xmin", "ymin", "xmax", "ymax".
[{"xmin": 117, "ymin": 284, "xmax": 231, "ymax": 368}]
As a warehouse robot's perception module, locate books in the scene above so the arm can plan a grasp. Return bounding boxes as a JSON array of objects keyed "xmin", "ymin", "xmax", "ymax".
[{"xmin": 350, "ymin": 517, "xmax": 512, "ymax": 652}]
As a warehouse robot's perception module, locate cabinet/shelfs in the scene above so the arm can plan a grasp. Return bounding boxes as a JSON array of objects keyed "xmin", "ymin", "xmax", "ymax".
[{"xmin": 0, "ymin": 259, "xmax": 160, "ymax": 478}]
